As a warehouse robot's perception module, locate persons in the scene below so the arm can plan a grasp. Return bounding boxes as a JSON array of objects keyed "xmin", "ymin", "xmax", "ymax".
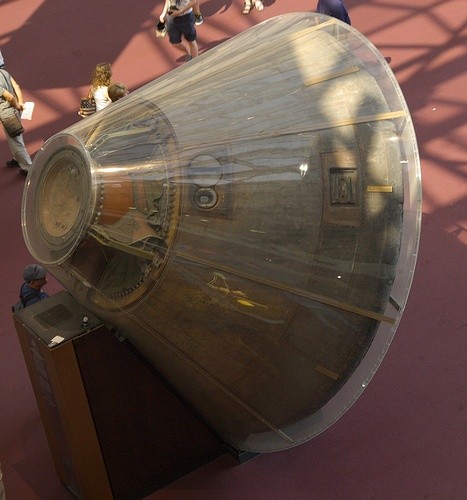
[
  {"xmin": 108, "ymin": 82, "xmax": 127, "ymax": 102},
  {"xmin": 242, "ymin": 0, "xmax": 264, "ymax": 15},
  {"xmin": 194, "ymin": 0, "xmax": 203, "ymax": 26},
  {"xmin": 154, "ymin": 0, "xmax": 198, "ymax": 63},
  {"xmin": 87, "ymin": 62, "xmax": 112, "ymax": 111},
  {"xmin": 0, "ymin": 70, "xmax": 33, "ymax": 175},
  {"xmin": 317, "ymin": 0, "xmax": 351, "ymax": 27},
  {"xmin": 12, "ymin": 264, "xmax": 50, "ymax": 309}
]
